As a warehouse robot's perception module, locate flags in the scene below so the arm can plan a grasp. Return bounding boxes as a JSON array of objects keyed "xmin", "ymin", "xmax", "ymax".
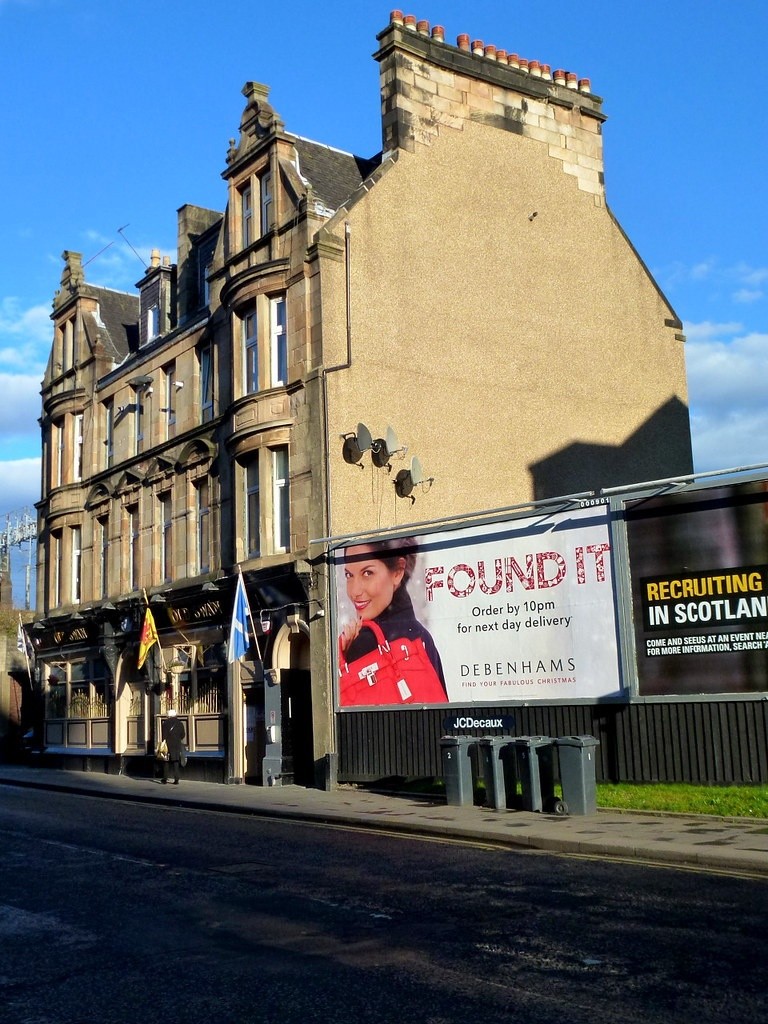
[
  {"xmin": 228, "ymin": 574, "xmax": 251, "ymax": 663},
  {"xmin": 18, "ymin": 623, "xmax": 34, "ymax": 659},
  {"xmin": 138, "ymin": 608, "xmax": 159, "ymax": 670}
]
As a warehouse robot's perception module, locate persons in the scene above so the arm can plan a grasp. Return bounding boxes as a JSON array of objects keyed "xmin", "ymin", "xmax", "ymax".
[
  {"xmin": 161, "ymin": 710, "xmax": 185, "ymax": 783},
  {"xmin": 335, "ymin": 536, "xmax": 449, "ymax": 705}
]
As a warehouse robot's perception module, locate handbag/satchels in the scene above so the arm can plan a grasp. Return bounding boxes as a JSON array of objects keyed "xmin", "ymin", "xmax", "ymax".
[
  {"xmin": 338, "ymin": 621, "xmax": 450, "ymax": 705},
  {"xmin": 156, "ymin": 741, "xmax": 170, "ymax": 761}
]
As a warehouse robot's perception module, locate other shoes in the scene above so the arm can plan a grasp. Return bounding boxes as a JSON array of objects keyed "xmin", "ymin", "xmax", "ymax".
[
  {"xmin": 173, "ymin": 780, "xmax": 179, "ymax": 784},
  {"xmin": 161, "ymin": 779, "xmax": 166, "ymax": 784}
]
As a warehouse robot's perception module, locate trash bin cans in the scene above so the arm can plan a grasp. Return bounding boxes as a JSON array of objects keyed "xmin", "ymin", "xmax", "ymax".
[
  {"xmin": 477, "ymin": 735, "xmax": 520, "ymax": 809},
  {"xmin": 553, "ymin": 734, "xmax": 601, "ymax": 816},
  {"xmin": 438, "ymin": 734, "xmax": 481, "ymax": 806},
  {"xmin": 512, "ymin": 735, "xmax": 561, "ymax": 813}
]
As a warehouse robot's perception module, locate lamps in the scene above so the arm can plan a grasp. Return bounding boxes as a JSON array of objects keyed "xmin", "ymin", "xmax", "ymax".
[
  {"xmin": 71, "ymin": 612, "xmax": 84, "ymax": 619},
  {"xmin": 168, "ymin": 660, "xmax": 186, "ymax": 674},
  {"xmin": 101, "ymin": 601, "xmax": 116, "ymax": 609},
  {"xmin": 150, "ymin": 594, "xmax": 166, "ymax": 602},
  {"xmin": 205, "ymin": 657, "xmax": 218, "ymax": 672},
  {"xmin": 33, "ymin": 623, "xmax": 46, "ymax": 629},
  {"xmin": 201, "ymin": 582, "xmax": 219, "ymax": 591}
]
